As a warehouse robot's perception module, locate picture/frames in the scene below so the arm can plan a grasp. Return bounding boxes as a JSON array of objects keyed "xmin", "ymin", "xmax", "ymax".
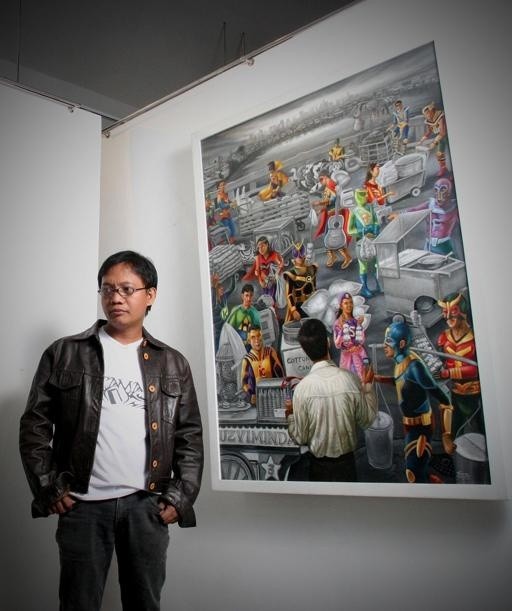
[{"xmin": 191, "ymin": 34, "xmax": 508, "ymax": 500}]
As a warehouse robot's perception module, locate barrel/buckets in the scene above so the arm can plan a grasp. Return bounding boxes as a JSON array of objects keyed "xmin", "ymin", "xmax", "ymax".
[
  {"xmin": 365, "ymin": 412, "xmax": 394, "ymax": 471},
  {"xmin": 452, "ymin": 434, "xmax": 490, "ymax": 484}
]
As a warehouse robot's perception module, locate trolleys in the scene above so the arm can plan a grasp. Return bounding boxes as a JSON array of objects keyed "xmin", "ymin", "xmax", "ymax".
[
  {"xmin": 381, "ymin": 145, "xmax": 429, "ymax": 205},
  {"xmin": 358, "ymin": 130, "xmax": 398, "ymax": 166}
]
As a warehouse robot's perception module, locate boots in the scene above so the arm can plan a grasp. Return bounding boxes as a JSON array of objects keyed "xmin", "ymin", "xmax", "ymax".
[
  {"xmin": 339, "ymin": 248, "xmax": 353, "ymax": 270},
  {"xmin": 372, "ymin": 272, "xmax": 384, "ymax": 294},
  {"xmin": 327, "ymin": 251, "xmax": 338, "ymax": 267},
  {"xmin": 436, "ymin": 153, "xmax": 448, "ymax": 176},
  {"xmin": 359, "ymin": 274, "xmax": 374, "ymax": 298}
]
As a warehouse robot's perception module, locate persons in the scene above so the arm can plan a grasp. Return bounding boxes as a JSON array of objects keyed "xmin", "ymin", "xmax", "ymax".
[{"xmin": 20, "ymin": 251, "xmax": 203, "ymax": 611}]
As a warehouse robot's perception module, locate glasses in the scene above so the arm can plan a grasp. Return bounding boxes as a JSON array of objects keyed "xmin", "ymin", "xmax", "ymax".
[{"xmin": 98, "ymin": 284, "xmax": 154, "ymax": 300}]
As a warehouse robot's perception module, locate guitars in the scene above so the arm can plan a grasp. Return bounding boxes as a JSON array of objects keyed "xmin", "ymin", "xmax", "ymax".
[{"xmin": 324, "ymin": 187, "xmax": 346, "ymax": 250}]
